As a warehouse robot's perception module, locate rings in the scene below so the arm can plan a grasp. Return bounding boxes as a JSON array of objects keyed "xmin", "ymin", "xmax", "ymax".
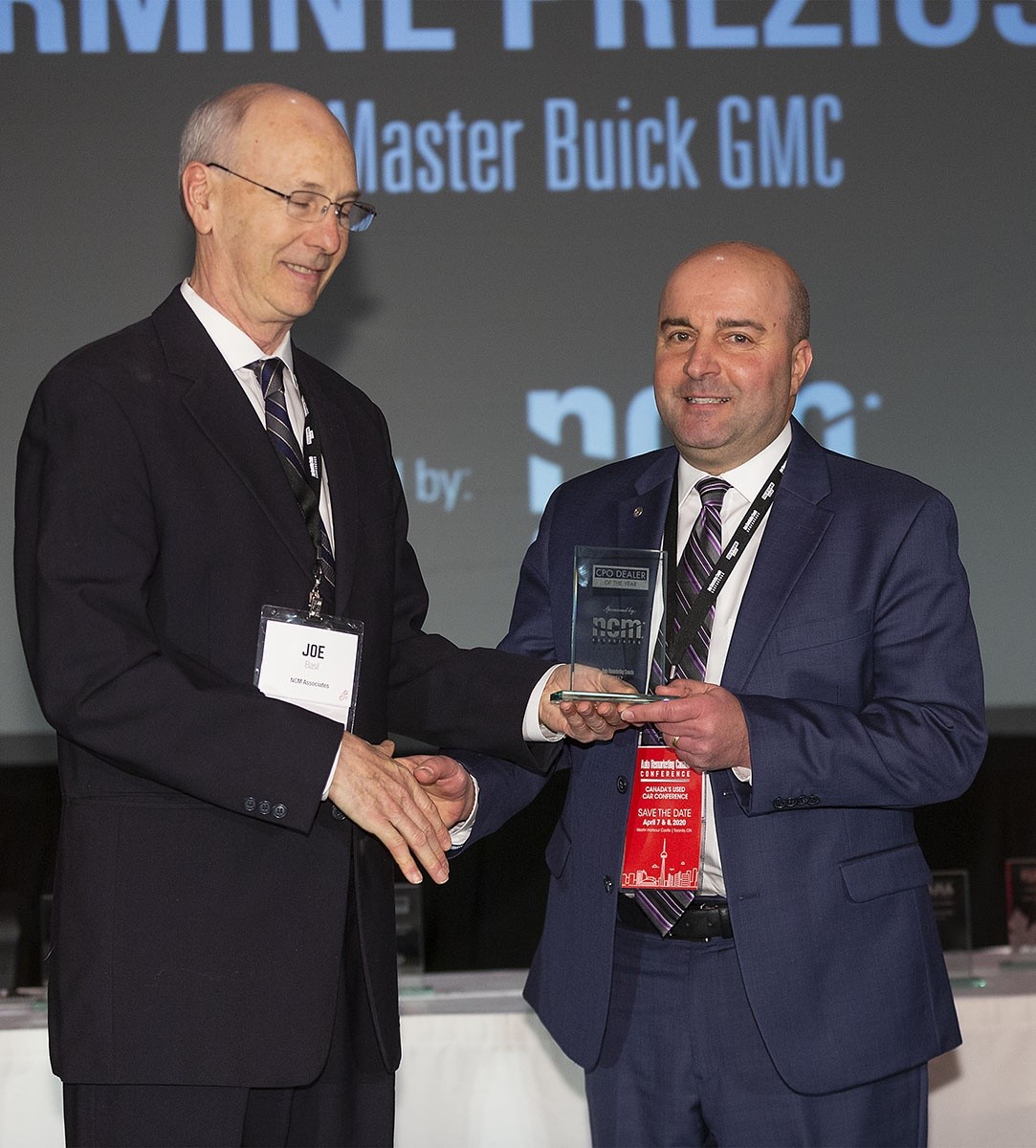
[{"xmin": 672, "ymin": 736, "xmax": 680, "ymax": 747}]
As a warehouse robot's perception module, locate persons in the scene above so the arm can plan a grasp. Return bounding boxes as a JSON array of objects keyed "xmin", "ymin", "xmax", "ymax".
[
  {"xmin": 13, "ymin": 84, "xmax": 641, "ymax": 1148},
  {"xmin": 392, "ymin": 243, "xmax": 988, "ymax": 1148}
]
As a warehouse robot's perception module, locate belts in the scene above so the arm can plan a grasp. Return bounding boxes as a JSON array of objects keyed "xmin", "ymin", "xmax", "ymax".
[{"xmin": 615, "ymin": 893, "xmax": 732, "ymax": 942}]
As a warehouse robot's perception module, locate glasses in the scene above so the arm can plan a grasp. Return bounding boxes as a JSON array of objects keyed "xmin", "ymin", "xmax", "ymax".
[{"xmin": 207, "ymin": 161, "xmax": 377, "ymax": 234}]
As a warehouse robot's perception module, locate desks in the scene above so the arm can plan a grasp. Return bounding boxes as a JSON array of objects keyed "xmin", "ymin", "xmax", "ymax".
[{"xmin": 1, "ymin": 946, "xmax": 1036, "ymax": 1148}]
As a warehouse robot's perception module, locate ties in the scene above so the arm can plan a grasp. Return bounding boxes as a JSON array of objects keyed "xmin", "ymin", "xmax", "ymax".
[
  {"xmin": 248, "ymin": 356, "xmax": 322, "ymax": 567},
  {"xmin": 630, "ymin": 478, "xmax": 730, "ymax": 936}
]
